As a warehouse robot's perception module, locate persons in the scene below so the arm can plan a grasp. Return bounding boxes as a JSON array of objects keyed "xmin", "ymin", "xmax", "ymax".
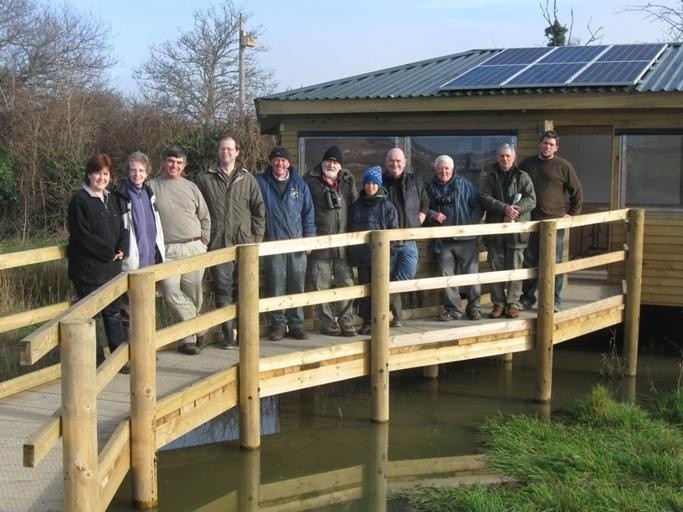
[
  {"xmin": 198, "ymin": 135, "xmax": 266, "ymax": 350},
  {"xmin": 516, "ymin": 130, "xmax": 583, "ymax": 312},
  {"xmin": 480, "ymin": 142, "xmax": 537, "ymax": 318},
  {"xmin": 426, "ymin": 154, "xmax": 484, "ymax": 318},
  {"xmin": 147, "ymin": 146, "xmax": 212, "ymax": 354},
  {"xmin": 255, "ymin": 146, "xmax": 317, "ymax": 341},
  {"xmin": 68, "ymin": 153, "xmax": 130, "ymax": 374},
  {"xmin": 382, "ymin": 147, "xmax": 430, "ymax": 327},
  {"xmin": 350, "ymin": 165, "xmax": 399, "ymax": 335},
  {"xmin": 303, "ymin": 145, "xmax": 356, "ymax": 336},
  {"xmin": 115, "ymin": 150, "xmax": 167, "ymax": 361}
]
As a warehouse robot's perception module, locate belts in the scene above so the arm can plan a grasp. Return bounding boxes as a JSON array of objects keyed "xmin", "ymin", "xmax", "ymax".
[{"xmin": 168, "ymin": 236, "xmax": 203, "ymax": 244}]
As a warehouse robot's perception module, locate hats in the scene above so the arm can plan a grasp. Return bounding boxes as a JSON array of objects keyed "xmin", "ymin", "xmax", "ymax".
[
  {"xmin": 360, "ymin": 165, "xmax": 384, "ymax": 187},
  {"xmin": 270, "ymin": 145, "xmax": 292, "ymax": 162},
  {"xmin": 323, "ymin": 144, "xmax": 343, "ymax": 164}
]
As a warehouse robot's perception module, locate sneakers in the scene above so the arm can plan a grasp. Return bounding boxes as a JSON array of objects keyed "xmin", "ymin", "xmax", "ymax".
[
  {"xmin": 436, "ymin": 309, "xmax": 465, "ymax": 321},
  {"xmin": 465, "ymin": 307, "xmax": 482, "ymax": 320},
  {"xmin": 287, "ymin": 326, "xmax": 310, "ymax": 340},
  {"xmin": 319, "ymin": 320, "xmax": 376, "ymax": 336},
  {"xmin": 269, "ymin": 325, "xmax": 286, "ymax": 341},
  {"xmin": 488, "ymin": 302, "xmax": 560, "ymax": 318},
  {"xmin": 177, "ymin": 329, "xmax": 240, "ymax": 354},
  {"xmin": 391, "ymin": 319, "xmax": 404, "ymax": 327}
]
